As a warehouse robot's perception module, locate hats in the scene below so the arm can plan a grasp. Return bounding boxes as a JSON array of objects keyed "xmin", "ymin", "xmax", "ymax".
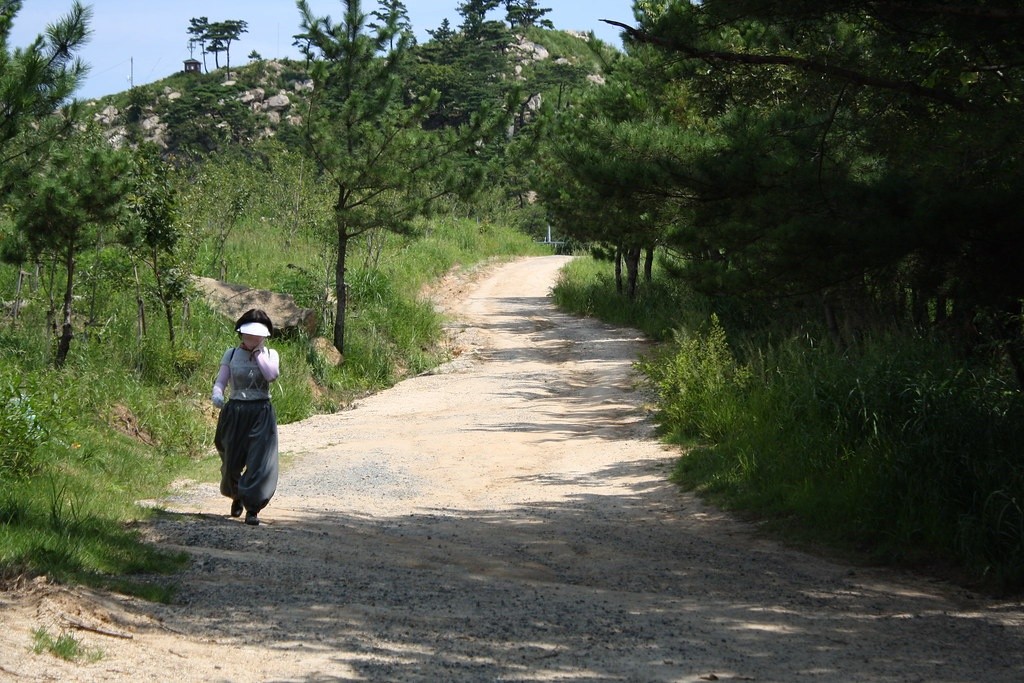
[{"xmin": 235, "ymin": 322, "xmax": 271, "ymax": 337}]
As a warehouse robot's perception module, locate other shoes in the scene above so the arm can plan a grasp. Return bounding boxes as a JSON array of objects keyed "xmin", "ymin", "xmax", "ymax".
[
  {"xmin": 245, "ymin": 512, "xmax": 259, "ymax": 525},
  {"xmin": 231, "ymin": 498, "xmax": 243, "ymax": 517}
]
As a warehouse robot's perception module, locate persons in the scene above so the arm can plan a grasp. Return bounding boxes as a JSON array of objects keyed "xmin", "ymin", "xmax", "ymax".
[{"xmin": 210, "ymin": 306, "xmax": 279, "ymax": 524}]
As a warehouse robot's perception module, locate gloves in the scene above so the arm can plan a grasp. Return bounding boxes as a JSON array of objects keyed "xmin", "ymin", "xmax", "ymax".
[{"xmin": 211, "ymin": 394, "xmax": 224, "ymax": 408}]
